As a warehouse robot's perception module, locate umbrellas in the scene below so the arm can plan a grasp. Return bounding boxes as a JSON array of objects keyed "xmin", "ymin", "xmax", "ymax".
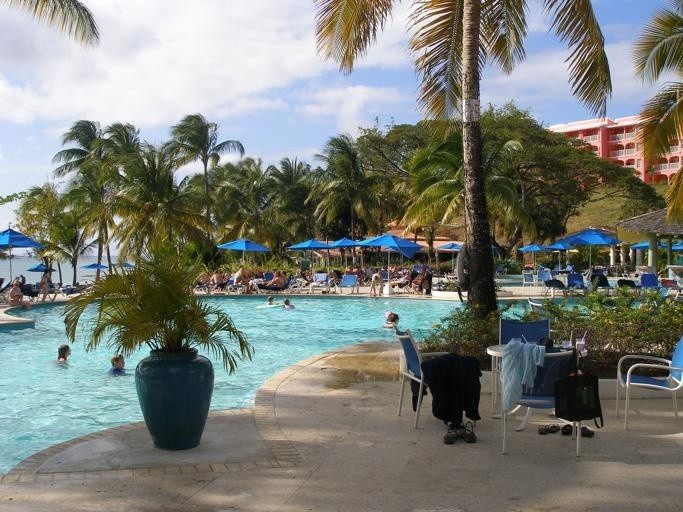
[
  {"xmin": 629, "ymin": 242, "xmax": 668, "ymax": 249},
  {"xmin": 327, "ymin": 237, "xmax": 361, "ymax": 264},
  {"xmin": 437, "ymin": 243, "xmax": 462, "ymax": 271},
  {"xmin": 217, "ymin": 238, "xmax": 270, "ymax": 264},
  {"xmin": 671, "ymin": 241, "xmax": 683, "ymax": 251},
  {"xmin": 564, "ymin": 226, "xmax": 622, "ymax": 269},
  {"xmin": 0, "ymin": 224, "xmax": 46, "ymax": 279},
  {"xmin": 385, "ymin": 247, "xmax": 419, "ymax": 264},
  {"xmin": 518, "ymin": 244, "xmax": 544, "ymax": 265},
  {"xmin": 545, "ymin": 238, "xmax": 575, "ymax": 269},
  {"xmin": 357, "ymin": 233, "xmax": 424, "ymax": 281},
  {"xmin": 287, "ymin": 239, "xmax": 335, "ymax": 265}
]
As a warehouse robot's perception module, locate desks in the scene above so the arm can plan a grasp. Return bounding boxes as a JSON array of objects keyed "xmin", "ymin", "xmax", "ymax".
[{"xmin": 486, "ymin": 341, "xmax": 588, "ymax": 433}]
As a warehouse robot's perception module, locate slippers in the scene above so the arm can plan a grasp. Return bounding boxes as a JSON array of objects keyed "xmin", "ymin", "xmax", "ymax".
[{"xmin": 539, "ymin": 424, "xmax": 594, "ymax": 437}]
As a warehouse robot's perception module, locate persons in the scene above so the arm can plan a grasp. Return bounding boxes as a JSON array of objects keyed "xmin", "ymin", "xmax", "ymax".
[
  {"xmin": 195, "ymin": 266, "xmax": 288, "ymax": 294},
  {"xmin": 267, "ymin": 296, "xmax": 273, "ymax": 305},
  {"xmin": 368, "ymin": 269, "xmax": 380, "ymax": 297},
  {"xmin": 55, "ymin": 344, "xmax": 72, "ymax": 365},
  {"xmin": 284, "ymin": 299, "xmax": 293, "ymax": 308},
  {"xmin": 346, "ymin": 266, "xmax": 369, "ymax": 281},
  {"xmin": 394, "ymin": 268, "xmax": 432, "ymax": 295},
  {"xmin": 384, "ymin": 312, "xmax": 398, "ymax": 331},
  {"xmin": 8, "ymin": 274, "xmax": 31, "ymax": 310},
  {"xmin": 302, "ymin": 268, "xmax": 312, "ymax": 282},
  {"xmin": 108, "ymin": 354, "xmax": 134, "ymax": 378},
  {"xmin": 328, "ymin": 272, "xmax": 339, "ymax": 286},
  {"xmin": 40, "ymin": 274, "xmax": 49, "ymax": 288}
]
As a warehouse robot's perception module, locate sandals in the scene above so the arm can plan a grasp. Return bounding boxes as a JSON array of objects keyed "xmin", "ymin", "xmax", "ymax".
[
  {"xmin": 456, "ymin": 422, "xmax": 475, "ymax": 442},
  {"xmin": 444, "ymin": 424, "xmax": 458, "ymax": 444}
]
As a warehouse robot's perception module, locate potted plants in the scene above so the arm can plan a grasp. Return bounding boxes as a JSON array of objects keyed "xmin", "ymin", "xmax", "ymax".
[{"xmin": 62, "ymin": 253, "xmax": 254, "ymax": 449}]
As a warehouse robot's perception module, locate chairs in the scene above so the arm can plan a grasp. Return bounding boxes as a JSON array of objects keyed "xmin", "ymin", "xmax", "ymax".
[
  {"xmin": 0, "ymin": 264, "xmax": 93, "ymax": 294},
  {"xmin": 441, "ymin": 265, "xmax": 682, "ymax": 294},
  {"xmin": 497, "ymin": 317, "xmax": 552, "ymax": 345},
  {"xmin": 501, "ymin": 350, "xmax": 581, "ymax": 457},
  {"xmin": 390, "ymin": 328, "xmax": 479, "ymax": 428},
  {"xmin": 191, "ymin": 265, "xmax": 442, "ymax": 295},
  {"xmin": 613, "ymin": 336, "xmax": 682, "ymax": 434}
]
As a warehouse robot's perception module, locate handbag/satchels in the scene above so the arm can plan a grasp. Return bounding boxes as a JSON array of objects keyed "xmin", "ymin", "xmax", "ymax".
[{"xmin": 554, "ymin": 349, "xmax": 602, "ymax": 422}]
